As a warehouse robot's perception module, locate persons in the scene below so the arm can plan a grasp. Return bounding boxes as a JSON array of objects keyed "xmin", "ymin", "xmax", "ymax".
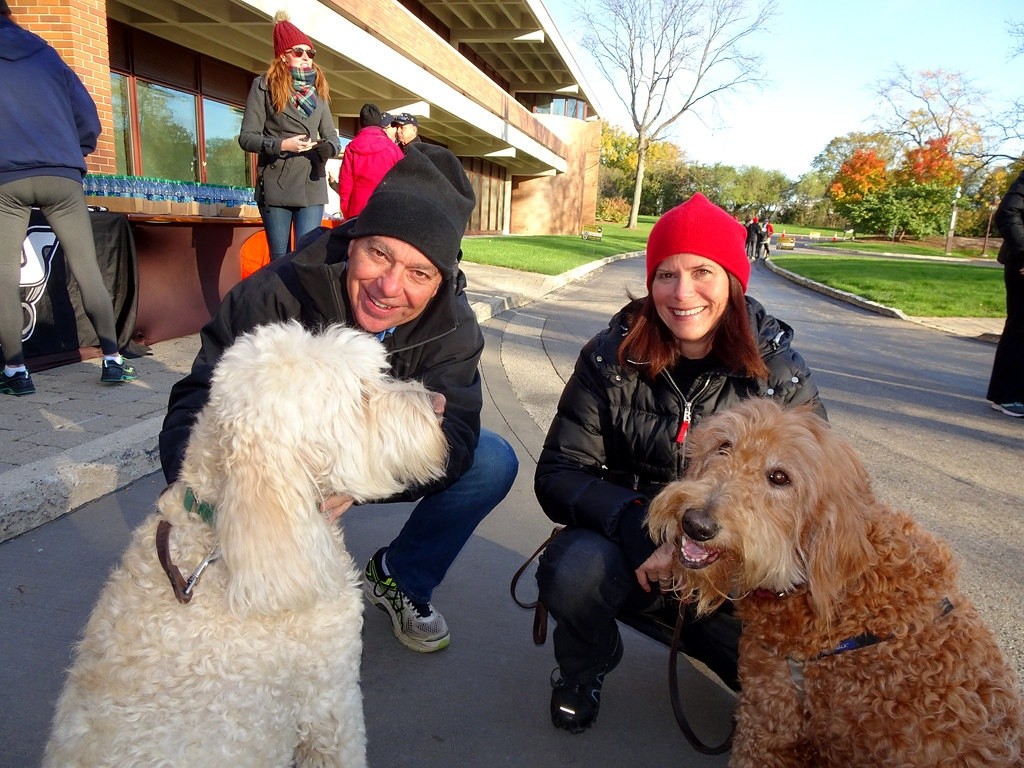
[
  {"xmin": 986, "ymin": 168, "xmax": 1024, "ymax": 418},
  {"xmin": 734, "ymin": 215, "xmax": 774, "ymax": 262},
  {"xmin": 533, "ymin": 193, "xmax": 830, "ymax": 733},
  {"xmin": 161, "ymin": 143, "xmax": 519, "ymax": 652},
  {"xmin": 0, "ymin": 1, "xmax": 136, "ymax": 395},
  {"xmin": 327, "ymin": 103, "xmax": 421, "ymax": 223},
  {"xmin": 238, "ymin": 10, "xmax": 342, "ymax": 265}
]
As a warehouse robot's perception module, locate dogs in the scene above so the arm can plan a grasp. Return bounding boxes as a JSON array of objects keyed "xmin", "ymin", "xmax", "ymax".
[
  {"xmin": 640, "ymin": 396, "xmax": 1024, "ymax": 768},
  {"xmin": 41, "ymin": 313, "xmax": 448, "ymax": 768}
]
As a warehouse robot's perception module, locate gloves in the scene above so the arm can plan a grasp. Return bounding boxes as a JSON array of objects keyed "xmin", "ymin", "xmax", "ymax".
[
  {"xmin": 307, "ymin": 150, "xmax": 321, "ymax": 180},
  {"xmin": 312, "ymin": 143, "xmax": 333, "ymax": 159}
]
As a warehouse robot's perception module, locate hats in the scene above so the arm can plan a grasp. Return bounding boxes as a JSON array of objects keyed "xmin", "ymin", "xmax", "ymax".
[
  {"xmin": 734, "ymin": 216, "xmax": 738, "ymax": 221},
  {"xmin": 753, "ymin": 218, "xmax": 758, "ymax": 223},
  {"xmin": 380, "ymin": 111, "xmax": 394, "ymax": 127},
  {"xmin": 392, "ymin": 113, "xmax": 418, "ymax": 128},
  {"xmin": 273, "ymin": 10, "xmax": 315, "ymax": 59},
  {"xmin": 347, "ymin": 142, "xmax": 476, "ymax": 276},
  {"xmin": 360, "ymin": 104, "xmax": 381, "ymax": 128},
  {"xmin": 646, "ymin": 193, "xmax": 750, "ymax": 292}
]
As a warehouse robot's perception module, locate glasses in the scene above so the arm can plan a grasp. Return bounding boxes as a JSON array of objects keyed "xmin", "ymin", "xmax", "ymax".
[{"xmin": 284, "ymin": 48, "xmax": 316, "ymax": 59}]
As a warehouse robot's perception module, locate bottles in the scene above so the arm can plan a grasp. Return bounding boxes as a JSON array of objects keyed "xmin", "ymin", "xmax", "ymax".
[{"xmin": 80, "ymin": 172, "xmax": 258, "ymax": 207}]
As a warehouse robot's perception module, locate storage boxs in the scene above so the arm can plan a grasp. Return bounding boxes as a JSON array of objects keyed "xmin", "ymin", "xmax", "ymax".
[{"xmin": 84, "ymin": 195, "xmax": 261, "ymax": 218}]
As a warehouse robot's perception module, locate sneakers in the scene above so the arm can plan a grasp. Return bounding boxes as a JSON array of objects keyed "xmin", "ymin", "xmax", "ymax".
[
  {"xmin": 100, "ymin": 358, "xmax": 138, "ymax": 384},
  {"xmin": 0, "ymin": 369, "xmax": 35, "ymax": 395},
  {"xmin": 356, "ymin": 546, "xmax": 450, "ymax": 652},
  {"xmin": 550, "ymin": 625, "xmax": 624, "ymax": 734},
  {"xmin": 991, "ymin": 401, "xmax": 1024, "ymax": 417}
]
